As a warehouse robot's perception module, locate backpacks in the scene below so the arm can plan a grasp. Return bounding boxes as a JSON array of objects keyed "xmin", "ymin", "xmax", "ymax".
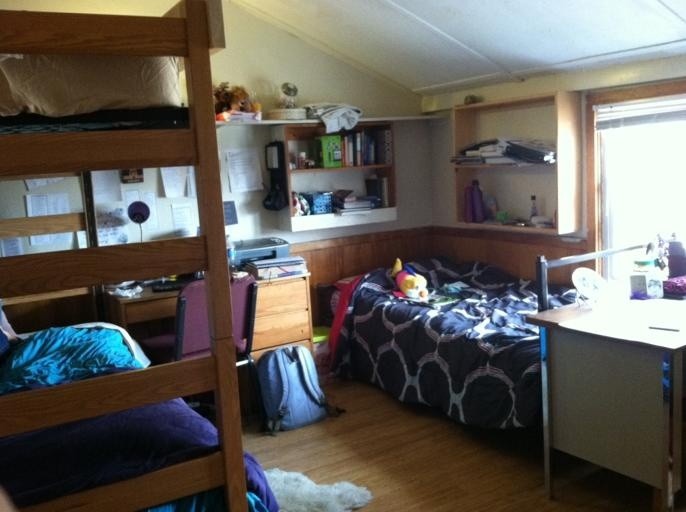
[{"xmin": 257, "ymin": 345, "xmax": 346, "ymax": 434}]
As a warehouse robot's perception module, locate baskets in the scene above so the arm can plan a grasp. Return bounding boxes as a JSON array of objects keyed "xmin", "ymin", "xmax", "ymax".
[{"xmin": 299, "ymin": 190, "xmax": 333, "ymax": 214}]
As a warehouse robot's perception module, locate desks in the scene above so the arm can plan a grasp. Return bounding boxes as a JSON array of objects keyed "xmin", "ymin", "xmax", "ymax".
[
  {"xmin": 526, "ymin": 262, "xmax": 686, "ymax": 511},
  {"xmin": 106, "ymin": 258, "xmax": 317, "ymax": 368}
]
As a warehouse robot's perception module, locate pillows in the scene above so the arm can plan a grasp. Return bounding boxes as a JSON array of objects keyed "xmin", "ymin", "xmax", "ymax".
[
  {"xmin": 0, "ymin": 49, "xmax": 184, "ymax": 119},
  {"xmin": 0, "ymin": 325, "xmax": 140, "ymax": 398}
]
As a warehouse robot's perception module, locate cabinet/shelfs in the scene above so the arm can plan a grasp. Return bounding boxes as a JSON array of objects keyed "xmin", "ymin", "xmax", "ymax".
[
  {"xmin": 449, "ymin": 89, "xmax": 582, "ymax": 236},
  {"xmin": 269, "ymin": 122, "xmax": 400, "ymax": 234}
]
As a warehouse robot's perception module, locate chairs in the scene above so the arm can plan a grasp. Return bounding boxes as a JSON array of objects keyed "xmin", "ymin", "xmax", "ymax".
[{"xmin": 129, "ymin": 270, "xmax": 258, "ymax": 428}]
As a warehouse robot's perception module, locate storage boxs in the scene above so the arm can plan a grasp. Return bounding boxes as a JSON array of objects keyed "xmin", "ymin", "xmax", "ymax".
[{"xmin": 311, "ymin": 326, "xmax": 335, "ymax": 374}]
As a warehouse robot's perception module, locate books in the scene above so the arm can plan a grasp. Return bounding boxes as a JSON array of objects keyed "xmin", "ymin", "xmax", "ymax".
[
  {"xmin": 244, "ymin": 255, "xmax": 307, "ymax": 280},
  {"xmin": 448, "ymin": 136, "xmax": 554, "ymax": 167},
  {"xmin": 315, "ymin": 130, "xmax": 396, "ymax": 216}
]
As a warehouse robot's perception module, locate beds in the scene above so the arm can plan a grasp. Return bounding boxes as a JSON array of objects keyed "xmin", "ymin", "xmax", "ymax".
[
  {"xmin": 0, "ymin": 1, "xmax": 278, "ymax": 512},
  {"xmin": 321, "ymin": 239, "xmax": 663, "ymax": 451}
]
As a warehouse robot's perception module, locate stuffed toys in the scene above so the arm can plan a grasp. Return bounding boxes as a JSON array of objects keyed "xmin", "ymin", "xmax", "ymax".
[
  {"xmin": 214, "ymin": 81, "xmax": 251, "ymax": 113},
  {"xmin": 388, "ymin": 256, "xmax": 429, "ymax": 299}
]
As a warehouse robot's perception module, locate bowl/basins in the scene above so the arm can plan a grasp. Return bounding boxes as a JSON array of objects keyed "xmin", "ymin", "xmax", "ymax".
[{"xmin": 265, "ymin": 108, "xmax": 307, "ymax": 122}]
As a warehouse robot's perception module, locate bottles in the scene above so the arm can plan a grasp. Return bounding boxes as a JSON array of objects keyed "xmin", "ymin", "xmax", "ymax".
[
  {"xmin": 464, "ymin": 187, "xmax": 473, "ymax": 223},
  {"xmin": 529, "ymin": 194, "xmax": 538, "ymax": 226},
  {"xmin": 471, "ymin": 180, "xmax": 485, "ymax": 222},
  {"xmin": 226, "ymin": 235, "xmax": 236, "ymax": 273}
]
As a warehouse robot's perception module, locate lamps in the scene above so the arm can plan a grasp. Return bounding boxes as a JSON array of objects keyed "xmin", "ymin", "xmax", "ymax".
[{"xmin": 127, "ymin": 201, "xmax": 151, "ymax": 243}]
